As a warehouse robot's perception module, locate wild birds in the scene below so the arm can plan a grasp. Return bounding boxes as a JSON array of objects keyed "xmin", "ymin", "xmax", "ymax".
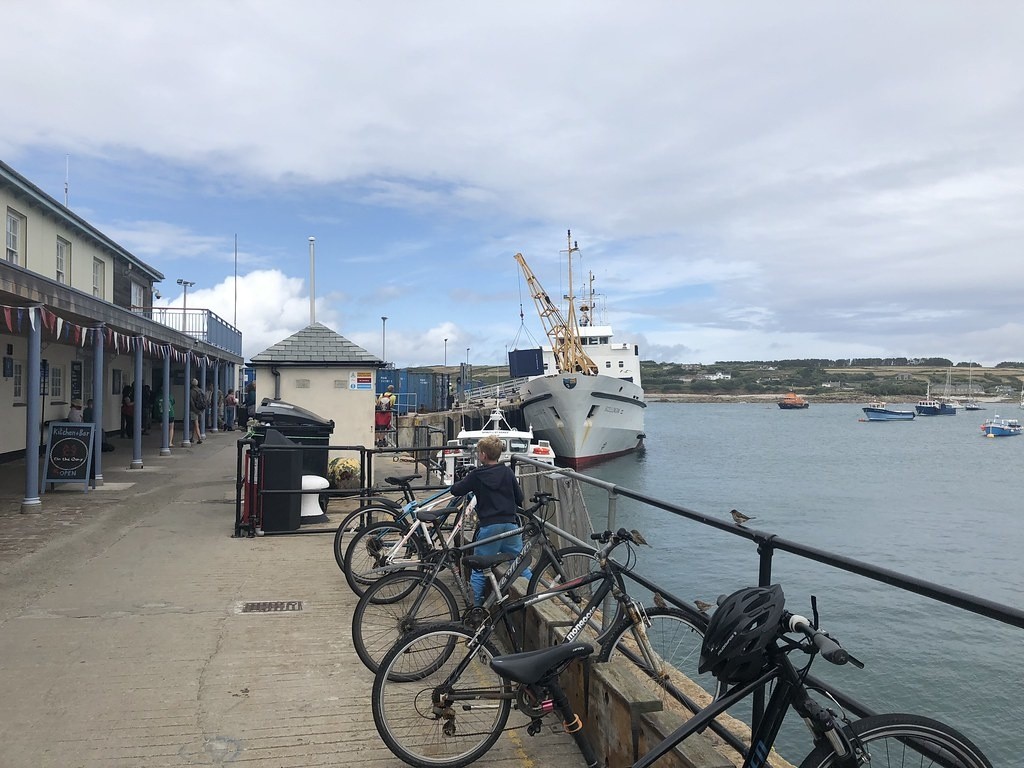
[
  {"xmin": 694, "ymin": 600, "xmax": 716, "ymax": 613},
  {"xmin": 630, "ymin": 530, "xmax": 651, "ymax": 549},
  {"xmin": 654, "ymin": 592, "xmax": 667, "ymax": 609},
  {"xmin": 730, "ymin": 509, "xmax": 756, "ymax": 526}
]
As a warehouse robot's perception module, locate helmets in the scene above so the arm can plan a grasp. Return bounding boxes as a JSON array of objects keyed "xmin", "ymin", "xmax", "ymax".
[{"xmin": 698, "ymin": 583, "xmax": 786, "ymax": 684}]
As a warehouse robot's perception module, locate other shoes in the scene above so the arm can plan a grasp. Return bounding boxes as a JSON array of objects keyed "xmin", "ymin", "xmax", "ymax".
[{"xmin": 223, "ymin": 424, "xmax": 228, "ymax": 431}]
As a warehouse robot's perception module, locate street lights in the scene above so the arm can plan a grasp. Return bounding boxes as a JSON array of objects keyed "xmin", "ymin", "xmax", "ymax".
[
  {"xmin": 308, "ymin": 236, "xmax": 316, "ymax": 325},
  {"xmin": 176, "ymin": 278, "xmax": 198, "ymax": 330},
  {"xmin": 381, "ymin": 316, "xmax": 391, "ymax": 361},
  {"xmin": 467, "ymin": 347, "xmax": 471, "ymax": 364},
  {"xmin": 444, "ymin": 338, "xmax": 448, "ymax": 365}
]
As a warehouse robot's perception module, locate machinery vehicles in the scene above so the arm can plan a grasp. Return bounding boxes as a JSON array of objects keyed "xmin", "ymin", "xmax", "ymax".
[{"xmin": 513, "ymin": 252, "xmax": 597, "ymax": 376}]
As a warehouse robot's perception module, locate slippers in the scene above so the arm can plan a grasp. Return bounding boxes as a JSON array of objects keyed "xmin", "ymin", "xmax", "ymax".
[{"xmin": 169, "ymin": 445, "xmax": 176, "ymax": 448}]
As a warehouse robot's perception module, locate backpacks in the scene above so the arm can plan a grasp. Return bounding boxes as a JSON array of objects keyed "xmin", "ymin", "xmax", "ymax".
[
  {"xmin": 157, "ymin": 398, "xmax": 172, "ymax": 413},
  {"xmin": 192, "ymin": 388, "xmax": 206, "ymax": 410},
  {"xmin": 379, "ymin": 393, "xmax": 393, "ymax": 410}
]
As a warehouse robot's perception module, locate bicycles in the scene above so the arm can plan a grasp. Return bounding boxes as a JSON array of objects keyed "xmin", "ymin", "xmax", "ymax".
[
  {"xmin": 491, "ymin": 586, "xmax": 997, "ymax": 768},
  {"xmin": 333, "ymin": 454, "xmax": 582, "ymax": 605},
  {"xmin": 368, "ymin": 525, "xmax": 727, "ymax": 768},
  {"xmin": 355, "ymin": 490, "xmax": 629, "ymax": 681}
]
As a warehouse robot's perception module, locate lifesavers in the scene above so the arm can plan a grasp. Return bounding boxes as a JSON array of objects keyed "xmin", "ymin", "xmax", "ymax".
[
  {"xmin": 532, "ymin": 448, "xmax": 550, "ymax": 455},
  {"xmin": 445, "ymin": 449, "xmax": 460, "ymax": 454}
]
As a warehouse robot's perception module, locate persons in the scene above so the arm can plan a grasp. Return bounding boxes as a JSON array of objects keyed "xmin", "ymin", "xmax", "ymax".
[
  {"xmin": 67, "ymin": 398, "xmax": 106, "ymax": 444},
  {"xmin": 190, "ymin": 378, "xmax": 202, "ymax": 444},
  {"xmin": 207, "ymin": 384, "xmax": 225, "ymax": 429},
  {"xmin": 375, "ymin": 385, "xmax": 396, "ymax": 447},
  {"xmin": 247, "ymin": 380, "xmax": 256, "ymax": 417},
  {"xmin": 225, "ymin": 389, "xmax": 237, "ymax": 431},
  {"xmin": 121, "ymin": 382, "xmax": 175, "ymax": 447},
  {"xmin": 451, "ymin": 436, "xmax": 533, "ymax": 605}
]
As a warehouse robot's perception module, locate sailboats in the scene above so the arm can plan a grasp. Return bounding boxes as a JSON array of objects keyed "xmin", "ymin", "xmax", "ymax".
[
  {"xmin": 1018, "ymin": 385, "xmax": 1024, "ymax": 410},
  {"xmin": 940, "ymin": 360, "xmax": 987, "ymax": 410}
]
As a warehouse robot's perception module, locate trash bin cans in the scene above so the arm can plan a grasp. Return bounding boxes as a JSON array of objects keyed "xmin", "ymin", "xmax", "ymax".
[
  {"xmin": 258, "ymin": 430, "xmax": 302, "ymax": 530},
  {"xmin": 253, "ymin": 398, "xmax": 335, "ymax": 513},
  {"xmin": 238, "ymin": 407, "xmax": 247, "ymax": 427}
]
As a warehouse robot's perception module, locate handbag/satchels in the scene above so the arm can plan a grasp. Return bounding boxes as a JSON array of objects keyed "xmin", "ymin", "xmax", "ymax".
[{"xmin": 121, "ymin": 403, "xmax": 136, "ymax": 417}]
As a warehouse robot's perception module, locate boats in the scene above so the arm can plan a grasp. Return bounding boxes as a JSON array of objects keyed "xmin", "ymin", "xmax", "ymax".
[
  {"xmin": 862, "ymin": 396, "xmax": 915, "ymax": 422},
  {"xmin": 981, "ymin": 408, "xmax": 1024, "ymax": 436},
  {"xmin": 915, "ymin": 383, "xmax": 956, "ymax": 416},
  {"xmin": 520, "ymin": 230, "xmax": 653, "ymax": 469},
  {"xmin": 777, "ymin": 384, "xmax": 809, "ymax": 411},
  {"xmin": 430, "ymin": 366, "xmax": 559, "ymax": 485}
]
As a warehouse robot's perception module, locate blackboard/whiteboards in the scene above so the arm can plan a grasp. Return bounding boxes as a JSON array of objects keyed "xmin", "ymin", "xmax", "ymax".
[{"xmin": 43, "ymin": 422, "xmax": 96, "ymax": 483}]
